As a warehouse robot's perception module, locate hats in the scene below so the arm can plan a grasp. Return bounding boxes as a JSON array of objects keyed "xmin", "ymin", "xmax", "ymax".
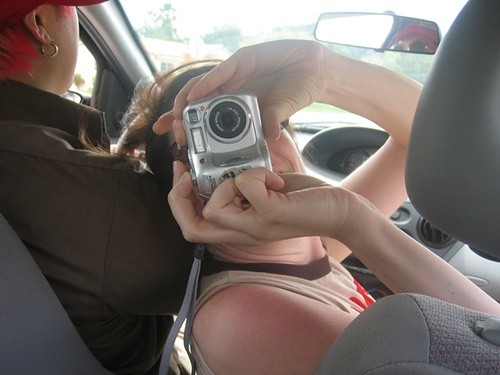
[
  {"xmin": 391, "ymin": 21, "xmax": 438, "ymax": 42},
  {"xmin": 0, "ymin": 0, "xmax": 109, "ymax": 32}
]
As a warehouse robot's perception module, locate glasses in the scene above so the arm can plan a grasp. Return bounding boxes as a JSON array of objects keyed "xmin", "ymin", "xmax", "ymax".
[{"xmin": 390, "ymin": 41, "xmax": 425, "ymax": 52}]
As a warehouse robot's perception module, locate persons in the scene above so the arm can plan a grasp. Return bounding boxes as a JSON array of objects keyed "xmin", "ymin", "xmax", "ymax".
[
  {"xmin": 390, "ymin": 21, "xmax": 439, "ymax": 55},
  {"xmin": 0, "ymin": 0, "xmax": 193, "ymax": 375},
  {"xmin": 112, "ymin": 38, "xmax": 500, "ymax": 375}
]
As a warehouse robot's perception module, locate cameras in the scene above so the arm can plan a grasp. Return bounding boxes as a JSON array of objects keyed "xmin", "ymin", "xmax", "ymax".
[{"xmin": 182, "ymin": 93, "xmax": 273, "ymax": 209}]
